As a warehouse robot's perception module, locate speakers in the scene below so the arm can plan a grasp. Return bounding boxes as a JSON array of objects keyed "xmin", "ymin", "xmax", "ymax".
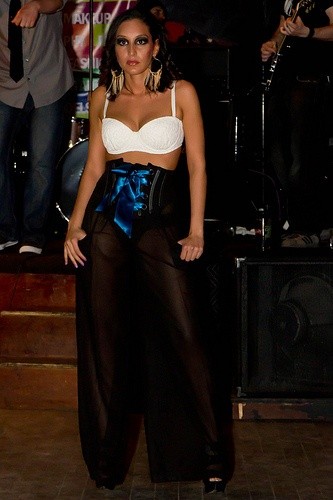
[{"xmin": 197, "ymin": 251, "xmax": 333, "ymax": 395}]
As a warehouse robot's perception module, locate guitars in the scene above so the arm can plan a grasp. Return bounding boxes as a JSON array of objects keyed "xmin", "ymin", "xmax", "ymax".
[{"xmin": 261, "ymin": 0, "xmax": 315, "ymax": 93}]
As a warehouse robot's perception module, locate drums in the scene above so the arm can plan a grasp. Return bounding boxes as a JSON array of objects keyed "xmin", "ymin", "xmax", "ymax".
[{"xmin": 53, "ymin": 138, "xmax": 88, "ymax": 223}]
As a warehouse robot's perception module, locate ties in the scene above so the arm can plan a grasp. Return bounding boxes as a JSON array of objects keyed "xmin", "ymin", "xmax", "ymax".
[{"xmin": 8, "ymin": 0, "xmax": 25, "ymax": 83}]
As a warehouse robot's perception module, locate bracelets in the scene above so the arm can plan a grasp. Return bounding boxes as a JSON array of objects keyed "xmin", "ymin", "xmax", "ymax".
[{"xmin": 306, "ymin": 26, "xmax": 315, "ymax": 39}]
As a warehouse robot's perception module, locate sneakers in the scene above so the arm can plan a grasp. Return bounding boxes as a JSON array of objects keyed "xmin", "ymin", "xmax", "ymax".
[{"xmin": 281, "ymin": 234, "xmax": 319, "ymax": 248}]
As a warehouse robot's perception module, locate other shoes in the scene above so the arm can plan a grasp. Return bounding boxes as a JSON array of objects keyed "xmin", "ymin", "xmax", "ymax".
[
  {"xmin": 18, "ymin": 241, "xmax": 43, "ymax": 255},
  {"xmin": 0, "ymin": 237, "xmax": 18, "ymax": 252},
  {"xmin": 95, "ymin": 474, "xmax": 115, "ymax": 490},
  {"xmin": 203, "ymin": 475, "xmax": 225, "ymax": 493}
]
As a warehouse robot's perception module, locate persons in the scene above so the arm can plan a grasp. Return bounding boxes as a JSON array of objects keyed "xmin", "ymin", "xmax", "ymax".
[
  {"xmin": 0, "ymin": 0, "xmax": 76, "ymax": 259},
  {"xmin": 60, "ymin": 8, "xmax": 227, "ymax": 496},
  {"xmin": 149, "ymin": 6, "xmax": 169, "ymax": 27},
  {"xmin": 259, "ymin": 0, "xmax": 333, "ymax": 249}
]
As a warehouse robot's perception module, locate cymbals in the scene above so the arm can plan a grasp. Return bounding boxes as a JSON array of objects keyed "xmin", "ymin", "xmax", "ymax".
[{"xmin": 73, "ymin": 69, "xmax": 101, "ymax": 78}]
